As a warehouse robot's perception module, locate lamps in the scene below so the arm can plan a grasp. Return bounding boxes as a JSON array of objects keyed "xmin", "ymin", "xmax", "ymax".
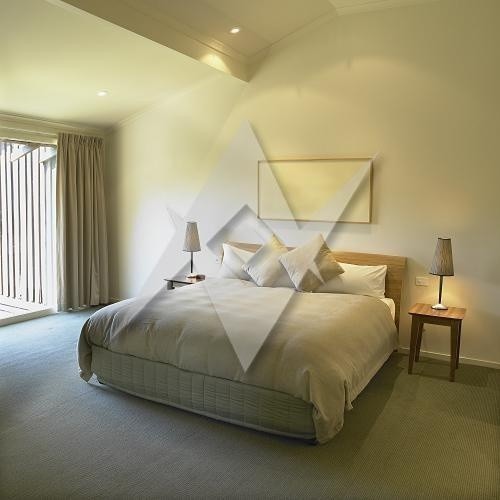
[
  {"xmin": 429, "ymin": 238, "xmax": 454, "ymax": 310},
  {"xmin": 181, "ymin": 222, "xmax": 201, "ymax": 273}
]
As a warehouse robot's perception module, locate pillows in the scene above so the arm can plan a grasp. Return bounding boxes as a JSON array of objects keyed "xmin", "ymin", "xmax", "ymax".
[{"xmin": 215, "ymin": 234, "xmax": 388, "ymax": 299}]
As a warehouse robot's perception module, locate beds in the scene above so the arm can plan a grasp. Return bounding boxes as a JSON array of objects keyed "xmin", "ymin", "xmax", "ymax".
[{"xmin": 78, "ymin": 240, "xmax": 407, "ymax": 446}]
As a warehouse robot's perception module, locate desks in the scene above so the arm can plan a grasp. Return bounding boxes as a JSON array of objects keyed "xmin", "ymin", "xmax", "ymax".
[
  {"xmin": 163, "ymin": 276, "xmax": 207, "ymax": 290},
  {"xmin": 408, "ymin": 303, "xmax": 467, "ymax": 382}
]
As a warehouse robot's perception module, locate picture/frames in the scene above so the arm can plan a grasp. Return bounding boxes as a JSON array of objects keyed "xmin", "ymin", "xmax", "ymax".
[{"xmin": 257, "ymin": 157, "xmax": 373, "ymax": 224}]
